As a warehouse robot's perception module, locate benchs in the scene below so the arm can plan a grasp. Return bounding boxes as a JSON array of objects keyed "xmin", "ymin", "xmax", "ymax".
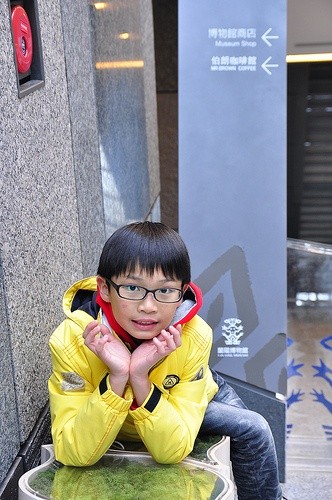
[{"xmin": 16, "ymin": 432, "xmax": 236, "ymax": 500}]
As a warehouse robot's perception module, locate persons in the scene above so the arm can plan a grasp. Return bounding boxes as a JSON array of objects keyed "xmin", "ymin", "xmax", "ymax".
[
  {"xmin": 45, "ymin": 221, "xmax": 286, "ymax": 500},
  {"xmin": 51, "ymin": 466, "xmax": 218, "ymax": 500}
]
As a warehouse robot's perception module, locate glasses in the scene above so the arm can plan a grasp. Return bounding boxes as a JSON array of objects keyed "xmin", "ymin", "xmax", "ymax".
[{"xmin": 107, "ymin": 278, "xmax": 184, "ymax": 304}]
